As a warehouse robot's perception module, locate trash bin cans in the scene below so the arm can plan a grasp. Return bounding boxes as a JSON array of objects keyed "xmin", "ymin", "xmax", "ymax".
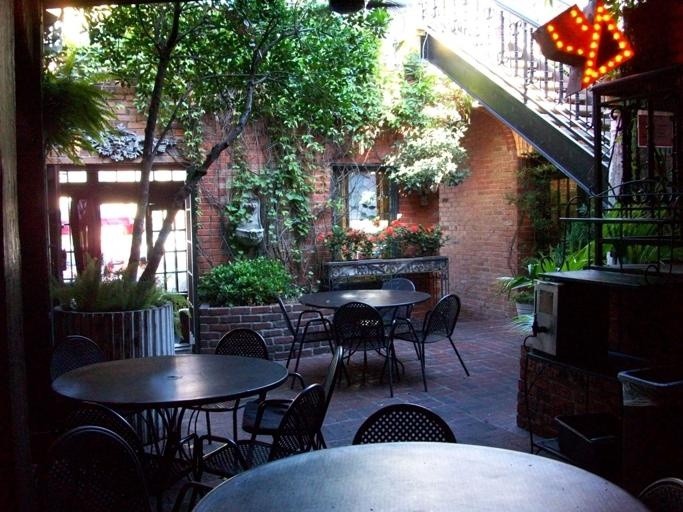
[{"xmin": 618, "ymin": 365, "xmax": 683, "ymax": 498}]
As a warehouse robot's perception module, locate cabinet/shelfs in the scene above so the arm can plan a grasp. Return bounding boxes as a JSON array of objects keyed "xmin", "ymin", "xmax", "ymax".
[{"xmin": 527, "ymin": 267, "xmax": 682, "ymax": 475}]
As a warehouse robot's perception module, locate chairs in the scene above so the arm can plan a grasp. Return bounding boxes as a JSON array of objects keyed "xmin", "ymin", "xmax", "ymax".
[
  {"xmin": 638, "ymin": 477, "xmax": 682, "ymax": 511},
  {"xmin": 61, "ymin": 402, "xmax": 161, "ymax": 510},
  {"xmin": 242, "ymin": 345, "xmax": 343, "ymax": 463},
  {"xmin": 54, "ymin": 335, "xmax": 104, "ymax": 379},
  {"xmin": 352, "ymin": 404, "xmax": 456, "ymax": 444},
  {"xmin": 332, "ymin": 301, "xmax": 401, "ymax": 398},
  {"xmin": 380, "ymin": 294, "xmax": 470, "ymax": 392},
  {"xmin": 174, "ymin": 383, "xmax": 326, "ymax": 512},
  {"xmin": 377, "ymin": 277, "xmax": 416, "ymax": 328},
  {"xmin": 44, "ymin": 425, "xmax": 153, "ymax": 510},
  {"xmin": 277, "ymin": 295, "xmax": 350, "ymax": 390},
  {"xmin": 186, "ymin": 327, "xmax": 270, "ymax": 444}
]
